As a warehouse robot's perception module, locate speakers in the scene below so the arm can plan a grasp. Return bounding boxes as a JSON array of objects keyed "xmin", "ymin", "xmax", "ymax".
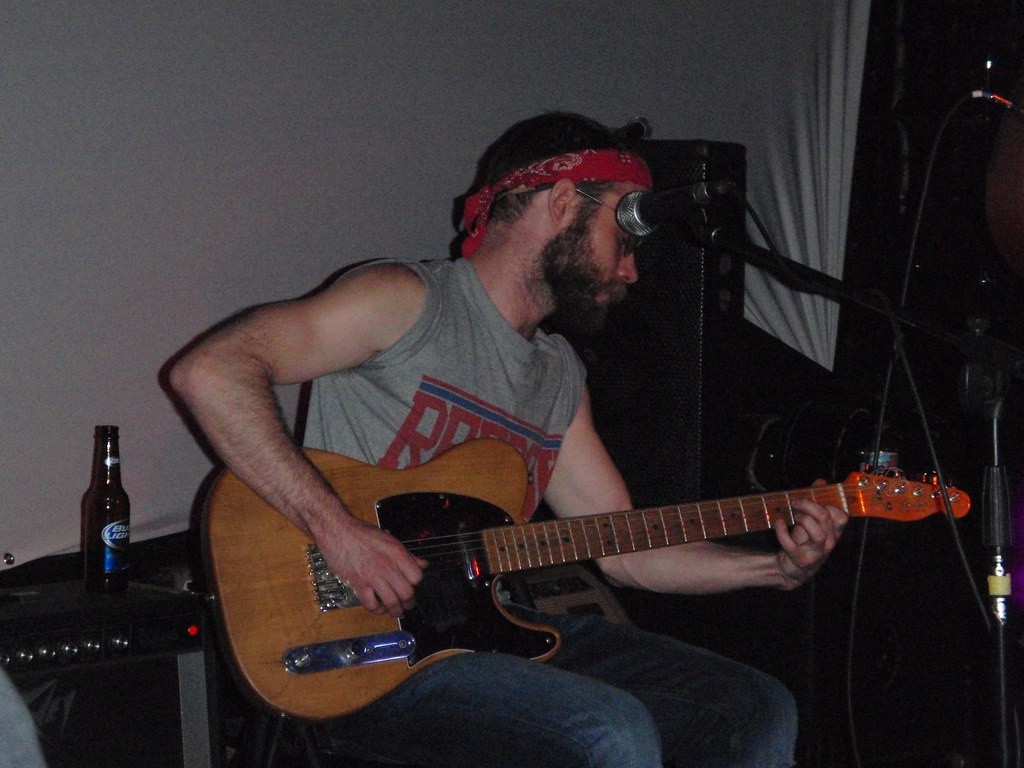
[
  {"xmin": 0, "ymin": 570, "xmax": 226, "ymax": 768},
  {"xmin": 536, "ymin": 139, "xmax": 749, "ymax": 511}
]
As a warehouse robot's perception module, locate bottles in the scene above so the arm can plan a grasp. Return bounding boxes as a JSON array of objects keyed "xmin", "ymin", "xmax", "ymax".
[{"xmin": 80, "ymin": 426, "xmax": 131, "ymax": 591}]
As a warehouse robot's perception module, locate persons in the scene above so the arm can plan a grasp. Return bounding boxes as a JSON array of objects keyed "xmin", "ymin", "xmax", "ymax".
[{"xmin": 168, "ymin": 112, "xmax": 847, "ymax": 768}]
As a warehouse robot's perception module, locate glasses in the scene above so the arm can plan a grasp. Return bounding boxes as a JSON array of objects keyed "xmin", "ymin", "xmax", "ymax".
[{"xmin": 576, "ymin": 188, "xmax": 649, "ymax": 257}]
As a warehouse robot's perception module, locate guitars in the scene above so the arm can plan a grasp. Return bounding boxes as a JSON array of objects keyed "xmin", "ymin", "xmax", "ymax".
[{"xmin": 191, "ymin": 434, "xmax": 973, "ymax": 721}]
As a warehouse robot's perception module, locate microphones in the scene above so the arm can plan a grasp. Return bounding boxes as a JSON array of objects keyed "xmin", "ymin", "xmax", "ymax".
[
  {"xmin": 617, "ymin": 117, "xmax": 651, "ymax": 140},
  {"xmin": 616, "ymin": 180, "xmax": 735, "ymax": 235}
]
